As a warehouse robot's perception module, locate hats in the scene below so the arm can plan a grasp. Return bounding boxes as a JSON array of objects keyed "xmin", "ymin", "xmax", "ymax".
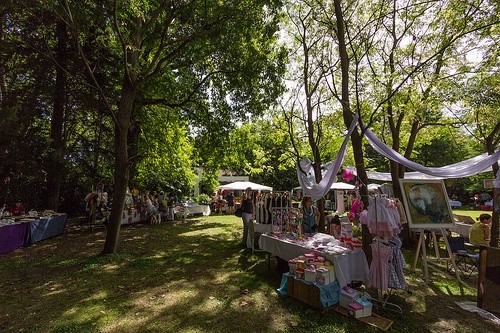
[{"xmin": 160, "ymin": 191, "xmax": 164, "ymax": 194}]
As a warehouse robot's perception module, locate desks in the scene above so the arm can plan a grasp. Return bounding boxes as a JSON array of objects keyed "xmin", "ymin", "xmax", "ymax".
[
  {"xmin": 456, "ymin": 223, "xmax": 473, "ymax": 237},
  {"xmin": 242, "ymin": 214, "xmax": 271, "ymax": 251},
  {"xmin": 188, "ymin": 205, "xmax": 212, "ymax": 216},
  {"xmin": 107, "ymin": 210, "xmax": 141, "ymax": 225},
  {"xmin": 259, "ymin": 232, "xmax": 371, "ymax": 289},
  {"xmin": 0, "ymin": 213, "xmax": 67, "ymax": 255}
]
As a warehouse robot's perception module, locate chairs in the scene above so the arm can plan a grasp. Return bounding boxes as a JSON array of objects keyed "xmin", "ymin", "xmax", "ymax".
[{"xmin": 445, "ymin": 229, "xmax": 481, "ymax": 279}]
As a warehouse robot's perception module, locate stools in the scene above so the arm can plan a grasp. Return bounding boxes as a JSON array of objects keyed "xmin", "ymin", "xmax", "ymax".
[{"xmin": 151, "ymin": 213, "xmax": 161, "ymax": 224}]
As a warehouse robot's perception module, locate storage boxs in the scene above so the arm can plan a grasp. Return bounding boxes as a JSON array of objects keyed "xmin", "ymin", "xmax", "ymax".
[
  {"xmin": 287, "ymin": 251, "xmax": 336, "ymax": 285},
  {"xmin": 339, "ymin": 286, "xmax": 373, "ymax": 318}
]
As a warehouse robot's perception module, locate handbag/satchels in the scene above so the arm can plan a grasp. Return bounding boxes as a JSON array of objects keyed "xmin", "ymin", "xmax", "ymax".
[
  {"xmin": 226, "ymin": 195, "xmax": 229, "ymax": 201},
  {"xmin": 234, "ymin": 198, "xmax": 244, "ymax": 217},
  {"xmin": 162, "ymin": 195, "xmax": 167, "ymax": 206}
]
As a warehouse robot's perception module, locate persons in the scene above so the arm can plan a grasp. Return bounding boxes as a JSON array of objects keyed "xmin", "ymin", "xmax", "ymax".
[
  {"xmin": 218, "ymin": 192, "xmax": 223, "ymax": 215},
  {"xmin": 211, "ymin": 192, "xmax": 216, "ymax": 210},
  {"xmin": 154, "ymin": 194, "xmax": 160, "ymax": 208},
  {"xmin": 166, "ymin": 194, "xmax": 177, "ymax": 221},
  {"xmin": 240, "ymin": 188, "xmax": 254, "ymax": 246},
  {"xmin": 228, "ymin": 194, "xmax": 236, "ymax": 214},
  {"xmin": 330, "ymin": 216, "xmax": 344, "ymax": 239},
  {"xmin": 470, "ymin": 213, "xmax": 492, "ymax": 247},
  {"xmin": 301, "ymin": 197, "xmax": 319, "ymax": 236}
]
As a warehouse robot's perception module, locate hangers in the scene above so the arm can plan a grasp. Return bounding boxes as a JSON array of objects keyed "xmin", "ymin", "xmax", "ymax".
[{"xmin": 369, "ymin": 235, "xmax": 387, "ymax": 246}]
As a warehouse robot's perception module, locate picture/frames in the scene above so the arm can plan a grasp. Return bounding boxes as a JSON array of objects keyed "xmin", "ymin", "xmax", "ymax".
[{"xmin": 398, "ymin": 178, "xmax": 456, "ymax": 229}]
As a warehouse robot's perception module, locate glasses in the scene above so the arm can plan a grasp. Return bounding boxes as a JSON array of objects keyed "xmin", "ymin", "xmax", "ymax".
[{"xmin": 307, "ymin": 201, "xmax": 313, "ymax": 203}]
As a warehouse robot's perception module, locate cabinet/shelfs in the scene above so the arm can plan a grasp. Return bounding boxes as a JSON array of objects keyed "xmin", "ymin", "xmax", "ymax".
[{"xmin": 287, "ymin": 276, "xmax": 337, "ymax": 312}]
